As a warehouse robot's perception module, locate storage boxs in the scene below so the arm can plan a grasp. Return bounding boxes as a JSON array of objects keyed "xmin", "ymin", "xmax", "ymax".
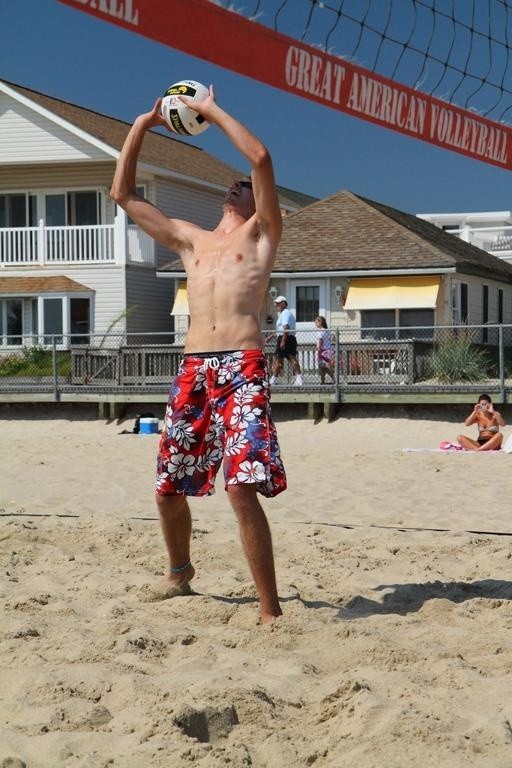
[{"xmin": 136, "ymin": 416, "xmax": 161, "ymax": 434}]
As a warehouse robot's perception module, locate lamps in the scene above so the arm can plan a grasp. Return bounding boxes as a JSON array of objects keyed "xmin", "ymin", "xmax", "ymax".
[
  {"xmin": 268, "ymin": 286, "xmax": 280, "ymax": 306},
  {"xmin": 331, "ymin": 285, "xmax": 345, "ymax": 306}
]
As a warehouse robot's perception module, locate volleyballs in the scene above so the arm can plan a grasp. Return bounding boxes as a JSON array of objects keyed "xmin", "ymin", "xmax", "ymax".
[{"xmin": 162, "ymin": 79, "xmax": 211, "ymax": 135}]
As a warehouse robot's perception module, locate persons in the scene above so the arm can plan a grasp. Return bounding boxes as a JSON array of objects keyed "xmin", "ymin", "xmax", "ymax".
[
  {"xmin": 455, "ymin": 394, "xmax": 505, "ymax": 450},
  {"xmin": 106, "ymin": 83, "xmax": 288, "ymax": 624},
  {"xmin": 314, "ymin": 317, "xmax": 335, "ymax": 383},
  {"xmin": 265, "ymin": 296, "xmax": 304, "ymax": 388}
]
